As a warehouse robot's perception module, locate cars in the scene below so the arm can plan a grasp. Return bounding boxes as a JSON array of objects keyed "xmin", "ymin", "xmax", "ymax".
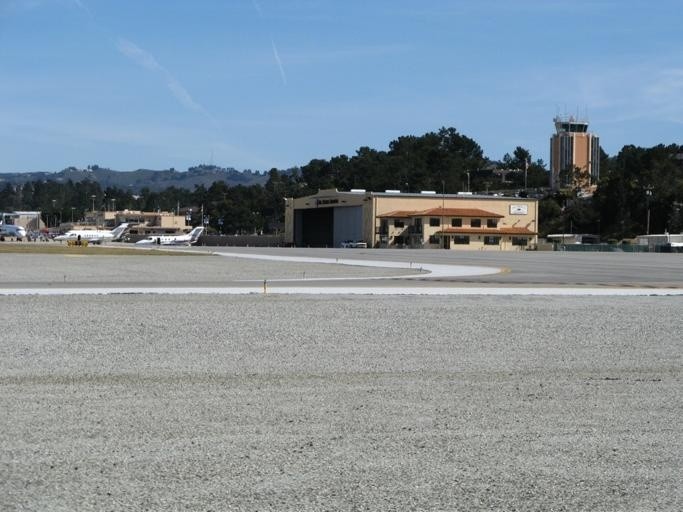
[
  {"xmin": 339, "ymin": 239, "xmax": 355, "ymax": 248},
  {"xmin": 475, "ymin": 187, "xmax": 585, "ymax": 198},
  {"xmin": 348, "ymin": 241, "xmax": 367, "ymax": 248}
]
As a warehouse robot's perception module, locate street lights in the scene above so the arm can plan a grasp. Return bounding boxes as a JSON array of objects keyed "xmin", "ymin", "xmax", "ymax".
[
  {"xmin": 466, "ymin": 169, "xmax": 470, "ymax": 192},
  {"xmin": 43, "ymin": 199, "xmax": 77, "ymax": 229},
  {"xmin": 405, "ymin": 182, "xmax": 409, "ymax": 193},
  {"xmin": 643, "ymin": 184, "xmax": 655, "ymax": 233},
  {"xmin": 91, "ymin": 195, "xmax": 96, "ymax": 210},
  {"xmin": 111, "ymin": 198, "xmax": 115, "ymax": 210},
  {"xmin": 251, "ymin": 211, "xmax": 258, "ymax": 236},
  {"xmin": 201, "ymin": 203, "xmax": 204, "ymax": 227},
  {"xmin": 523, "ymin": 157, "xmax": 527, "ymax": 191},
  {"xmin": 438, "ymin": 179, "xmax": 444, "ymax": 249}
]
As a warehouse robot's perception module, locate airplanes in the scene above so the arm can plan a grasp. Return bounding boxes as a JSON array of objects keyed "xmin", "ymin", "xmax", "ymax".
[
  {"xmin": 134, "ymin": 226, "xmax": 203, "ymax": 246},
  {"xmin": 53, "ymin": 223, "xmax": 128, "ymax": 245},
  {"xmin": 0, "ymin": 220, "xmax": 27, "ymax": 242}
]
